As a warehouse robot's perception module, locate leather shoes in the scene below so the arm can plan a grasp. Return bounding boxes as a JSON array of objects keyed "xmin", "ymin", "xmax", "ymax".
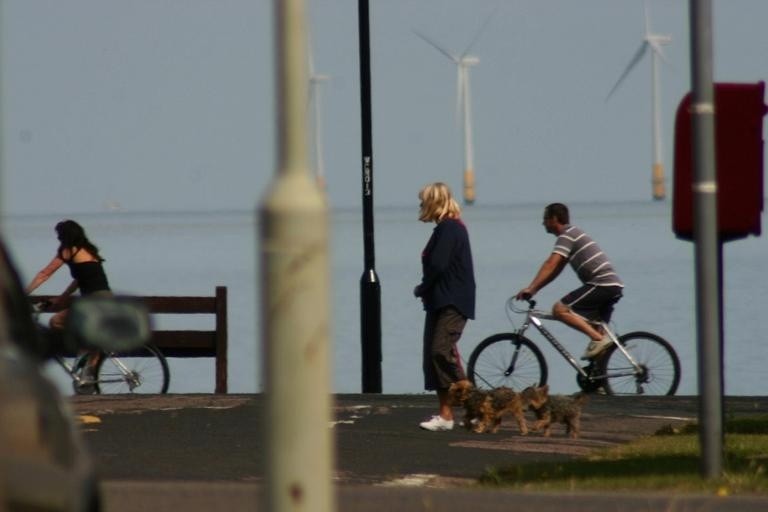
[{"xmin": 581, "ymin": 335, "xmax": 612, "ymax": 362}]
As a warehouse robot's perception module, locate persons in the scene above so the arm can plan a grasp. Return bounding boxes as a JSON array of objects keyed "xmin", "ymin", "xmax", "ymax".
[
  {"xmin": 414, "ymin": 182, "xmax": 476, "ymax": 432},
  {"xmin": 22, "ymin": 218, "xmax": 114, "ymax": 371},
  {"xmin": 515, "ymin": 202, "xmax": 624, "ymax": 395}
]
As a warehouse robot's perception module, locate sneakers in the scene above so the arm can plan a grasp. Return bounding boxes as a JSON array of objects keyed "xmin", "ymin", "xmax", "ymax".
[{"xmin": 419, "ymin": 415, "xmax": 455, "ymax": 432}]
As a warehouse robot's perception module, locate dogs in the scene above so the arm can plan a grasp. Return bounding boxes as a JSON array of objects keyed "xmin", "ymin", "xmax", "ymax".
[
  {"xmin": 445, "ymin": 379, "xmax": 530, "ymax": 436},
  {"xmin": 524, "ymin": 381, "xmax": 588, "ymax": 439}
]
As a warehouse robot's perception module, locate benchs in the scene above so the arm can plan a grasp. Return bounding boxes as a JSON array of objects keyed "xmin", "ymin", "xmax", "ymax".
[{"xmin": 24, "ymin": 285, "xmax": 230, "ymax": 395}]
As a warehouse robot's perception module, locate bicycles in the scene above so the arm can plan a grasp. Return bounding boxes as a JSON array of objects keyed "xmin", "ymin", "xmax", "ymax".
[
  {"xmin": 467, "ymin": 292, "xmax": 682, "ymax": 396},
  {"xmin": 27, "ymin": 298, "xmax": 170, "ymax": 396}
]
